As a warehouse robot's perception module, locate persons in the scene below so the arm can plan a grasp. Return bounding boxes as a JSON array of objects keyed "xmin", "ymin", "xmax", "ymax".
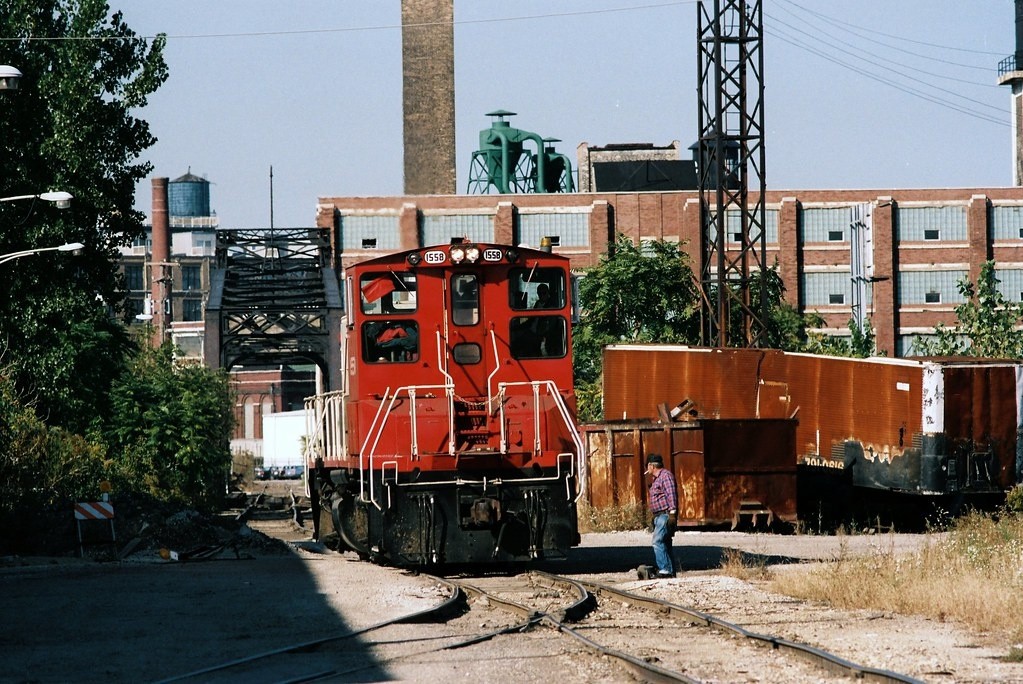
[
  {"xmin": 647, "ymin": 454, "xmax": 679, "ymax": 579},
  {"xmin": 523, "ymin": 283, "xmax": 552, "ymax": 336}
]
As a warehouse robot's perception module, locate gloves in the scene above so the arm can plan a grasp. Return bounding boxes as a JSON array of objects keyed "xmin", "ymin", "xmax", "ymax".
[{"xmin": 667, "ymin": 513, "xmax": 677, "ymax": 528}]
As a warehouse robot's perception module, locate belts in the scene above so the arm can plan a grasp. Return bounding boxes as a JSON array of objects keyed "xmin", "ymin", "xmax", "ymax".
[{"xmin": 654, "ymin": 511, "xmax": 666, "ymax": 516}]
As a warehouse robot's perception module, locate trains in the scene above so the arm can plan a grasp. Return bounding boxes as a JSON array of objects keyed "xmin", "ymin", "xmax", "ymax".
[{"xmin": 304, "ymin": 234, "xmax": 587, "ymax": 578}]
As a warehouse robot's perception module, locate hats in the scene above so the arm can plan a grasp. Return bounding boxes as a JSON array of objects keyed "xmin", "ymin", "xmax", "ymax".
[{"xmin": 644, "ymin": 454, "xmax": 662, "ymax": 466}]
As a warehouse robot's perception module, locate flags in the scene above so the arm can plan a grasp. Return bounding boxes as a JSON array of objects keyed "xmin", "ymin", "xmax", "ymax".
[{"xmin": 362, "ymin": 272, "xmax": 397, "ymax": 303}]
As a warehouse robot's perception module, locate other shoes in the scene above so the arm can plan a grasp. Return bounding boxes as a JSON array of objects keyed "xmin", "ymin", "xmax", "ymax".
[{"xmin": 650, "ymin": 571, "xmax": 675, "ymax": 578}]
